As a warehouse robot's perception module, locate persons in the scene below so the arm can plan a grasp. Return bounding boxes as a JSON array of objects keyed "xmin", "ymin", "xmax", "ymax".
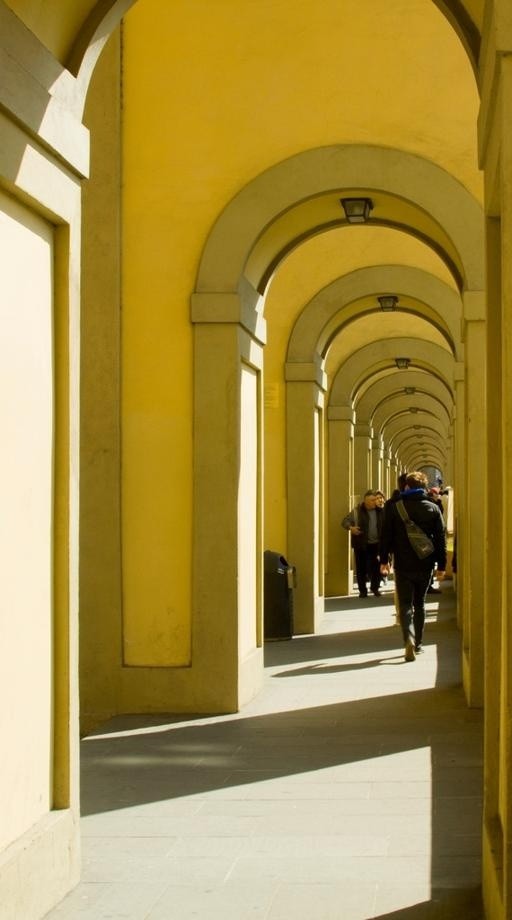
[{"xmin": 342, "ymin": 471, "xmax": 454, "ymax": 662}]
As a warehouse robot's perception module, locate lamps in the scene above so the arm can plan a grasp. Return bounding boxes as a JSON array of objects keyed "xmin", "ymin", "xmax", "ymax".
[
  {"xmin": 377, "ymin": 295, "xmax": 398, "ymax": 312},
  {"xmin": 340, "ymin": 198, "xmax": 373, "ymax": 224},
  {"xmin": 395, "ymin": 358, "xmax": 410, "ymax": 369},
  {"xmin": 404, "ymin": 387, "xmax": 429, "ymax": 463}
]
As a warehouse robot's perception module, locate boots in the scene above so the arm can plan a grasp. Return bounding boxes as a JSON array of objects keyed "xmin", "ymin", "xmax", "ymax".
[
  {"xmin": 359, "ymin": 587, "xmax": 367, "ymax": 598},
  {"xmin": 371, "ymin": 584, "xmax": 380, "ymax": 598}
]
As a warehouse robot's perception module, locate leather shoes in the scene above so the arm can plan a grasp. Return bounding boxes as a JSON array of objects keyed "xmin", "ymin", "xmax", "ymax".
[{"xmin": 426, "ymin": 584, "xmax": 443, "ymax": 595}]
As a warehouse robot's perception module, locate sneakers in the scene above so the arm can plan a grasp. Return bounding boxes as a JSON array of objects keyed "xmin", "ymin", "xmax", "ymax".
[{"xmin": 405, "ymin": 634, "xmax": 426, "ymax": 663}]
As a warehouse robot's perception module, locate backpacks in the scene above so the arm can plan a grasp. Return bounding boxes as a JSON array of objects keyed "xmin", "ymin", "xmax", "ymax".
[{"xmin": 403, "ymin": 520, "xmax": 437, "ymax": 562}]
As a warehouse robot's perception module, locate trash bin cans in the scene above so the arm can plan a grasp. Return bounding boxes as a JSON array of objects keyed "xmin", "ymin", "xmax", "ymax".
[{"xmin": 264, "ymin": 550, "xmax": 297, "ymax": 642}]
